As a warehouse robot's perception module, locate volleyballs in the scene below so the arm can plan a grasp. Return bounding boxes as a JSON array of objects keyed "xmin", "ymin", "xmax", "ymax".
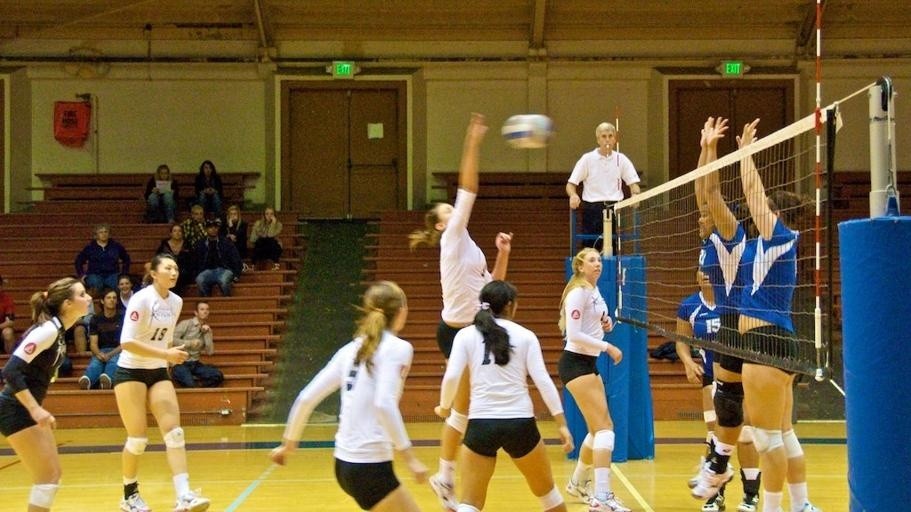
[{"xmin": 502, "ymin": 114, "xmax": 552, "ymax": 149}]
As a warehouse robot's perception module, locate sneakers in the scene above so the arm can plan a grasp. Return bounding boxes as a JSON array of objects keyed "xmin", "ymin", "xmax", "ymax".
[
  {"xmin": 79, "ymin": 376, "xmax": 90, "ymax": 390},
  {"xmin": 429, "ymin": 472, "xmax": 459, "ymax": 511},
  {"xmin": 702, "ymin": 494, "xmax": 726, "ymax": 511},
  {"xmin": 589, "ymin": 492, "xmax": 632, "ymax": 512},
  {"xmin": 100, "ymin": 374, "xmax": 111, "ymax": 389},
  {"xmin": 173, "ymin": 488, "xmax": 210, "ymax": 512},
  {"xmin": 120, "ymin": 492, "xmax": 150, "ymax": 511},
  {"xmin": 691, "ymin": 461, "xmax": 734, "ymax": 500},
  {"xmin": 737, "ymin": 493, "xmax": 760, "ymax": 511},
  {"xmin": 688, "ymin": 456, "xmax": 708, "ymax": 489},
  {"xmin": 565, "ymin": 478, "xmax": 594, "ymax": 505}
]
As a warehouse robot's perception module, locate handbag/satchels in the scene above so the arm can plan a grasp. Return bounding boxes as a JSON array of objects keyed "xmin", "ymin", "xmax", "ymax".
[{"xmin": 650, "ymin": 342, "xmax": 680, "ymax": 360}]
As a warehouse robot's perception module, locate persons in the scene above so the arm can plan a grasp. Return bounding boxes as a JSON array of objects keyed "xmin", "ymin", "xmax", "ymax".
[
  {"xmin": 59, "ymin": 222, "xmax": 224, "ymax": 388},
  {"xmin": 676, "ymin": 268, "xmax": 762, "ymax": 511},
  {"xmin": 686, "ymin": 117, "xmax": 755, "ymax": 501},
  {"xmin": 0, "ymin": 296, "xmax": 16, "ymax": 354},
  {"xmin": 0, "ymin": 276, "xmax": 93, "ymax": 512},
  {"xmin": 144, "ymin": 164, "xmax": 179, "ymax": 224},
  {"xmin": 737, "ymin": 116, "xmax": 815, "ymax": 510},
  {"xmin": 157, "ymin": 205, "xmax": 282, "ymax": 297},
  {"xmin": 434, "ymin": 280, "xmax": 576, "ymax": 512},
  {"xmin": 114, "ymin": 253, "xmax": 210, "ymax": 512},
  {"xmin": 194, "ymin": 160, "xmax": 226, "ymax": 226},
  {"xmin": 408, "ymin": 111, "xmax": 514, "ymax": 511},
  {"xmin": 269, "ymin": 280, "xmax": 431, "ymax": 512},
  {"xmin": 566, "ymin": 120, "xmax": 642, "ymax": 255},
  {"xmin": 557, "ymin": 248, "xmax": 623, "ymax": 511}
]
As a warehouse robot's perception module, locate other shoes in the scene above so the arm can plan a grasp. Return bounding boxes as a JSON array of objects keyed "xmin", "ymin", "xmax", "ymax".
[{"xmin": 799, "ymin": 502, "xmax": 817, "ymax": 511}]
